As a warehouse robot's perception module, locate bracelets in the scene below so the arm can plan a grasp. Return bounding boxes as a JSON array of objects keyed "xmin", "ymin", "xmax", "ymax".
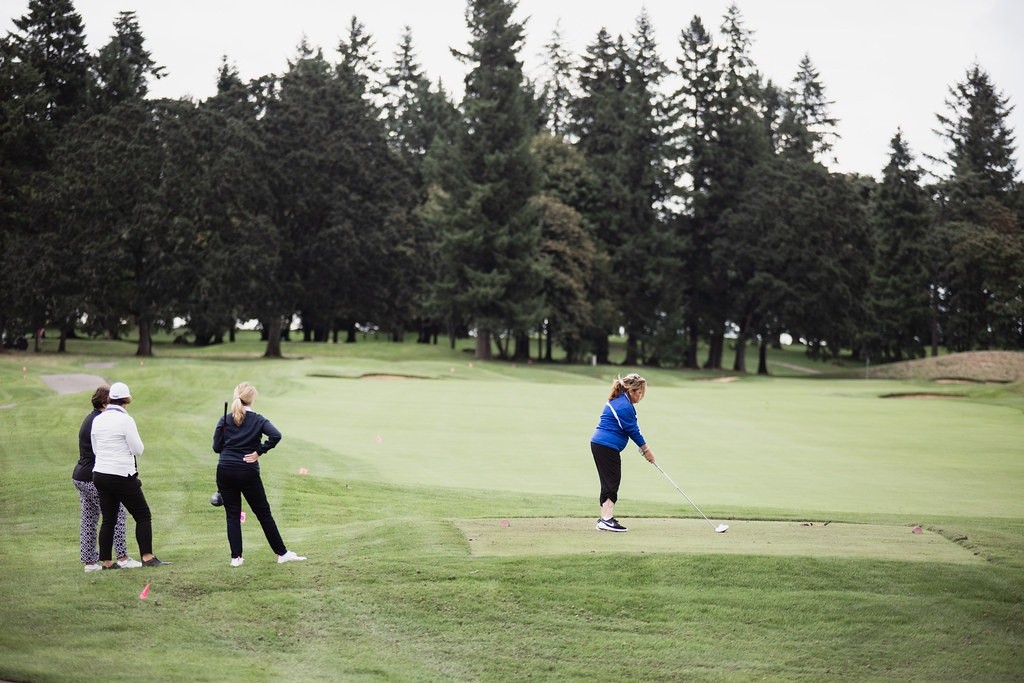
[{"xmin": 644, "ymin": 447, "xmax": 649, "ymax": 452}]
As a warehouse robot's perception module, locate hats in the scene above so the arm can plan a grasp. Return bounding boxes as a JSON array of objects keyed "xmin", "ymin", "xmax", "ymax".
[{"xmin": 109, "ymin": 382, "xmax": 130, "ymax": 399}]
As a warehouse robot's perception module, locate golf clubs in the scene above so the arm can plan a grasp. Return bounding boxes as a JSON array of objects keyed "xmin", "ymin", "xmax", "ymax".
[
  {"xmin": 637, "ymin": 447, "xmax": 730, "ymax": 533},
  {"xmin": 210, "ymin": 402, "xmax": 228, "ymax": 507}
]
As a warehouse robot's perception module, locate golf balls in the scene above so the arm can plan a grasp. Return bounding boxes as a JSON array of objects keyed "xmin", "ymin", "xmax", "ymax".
[{"xmin": 719, "ymin": 523, "xmax": 724, "ymax": 527}]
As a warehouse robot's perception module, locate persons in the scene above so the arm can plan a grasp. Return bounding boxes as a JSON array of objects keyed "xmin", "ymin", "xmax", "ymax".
[
  {"xmin": 72, "ymin": 386, "xmax": 142, "ymax": 572},
  {"xmin": 90, "ymin": 381, "xmax": 171, "ymax": 570},
  {"xmin": 591, "ymin": 374, "xmax": 655, "ymax": 532},
  {"xmin": 212, "ymin": 381, "xmax": 307, "ymax": 566}
]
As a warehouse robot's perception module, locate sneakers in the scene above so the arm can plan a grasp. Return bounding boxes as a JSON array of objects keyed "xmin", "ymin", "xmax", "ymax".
[
  {"xmin": 231, "ymin": 558, "xmax": 243, "ymax": 566},
  {"xmin": 597, "ymin": 517, "xmax": 628, "ymax": 532},
  {"xmin": 102, "ymin": 562, "xmax": 121, "ymax": 569},
  {"xmin": 117, "ymin": 557, "xmax": 143, "ymax": 568},
  {"xmin": 85, "ymin": 564, "xmax": 102, "ymax": 572},
  {"xmin": 142, "ymin": 556, "xmax": 172, "ymax": 567},
  {"xmin": 278, "ymin": 550, "xmax": 307, "ymax": 563}
]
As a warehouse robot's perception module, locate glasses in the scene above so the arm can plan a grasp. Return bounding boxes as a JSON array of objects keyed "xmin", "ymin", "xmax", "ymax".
[{"xmin": 631, "ymin": 375, "xmax": 639, "ymax": 384}]
